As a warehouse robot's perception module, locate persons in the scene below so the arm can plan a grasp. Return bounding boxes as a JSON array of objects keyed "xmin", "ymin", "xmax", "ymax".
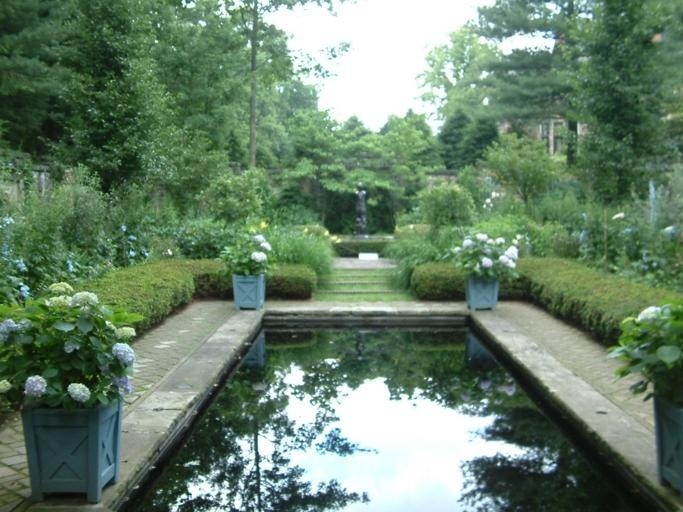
[
  {"xmin": 354, "ymin": 183, "xmax": 367, "ymax": 235},
  {"xmin": 356, "ymin": 334, "xmax": 365, "ymax": 361}
]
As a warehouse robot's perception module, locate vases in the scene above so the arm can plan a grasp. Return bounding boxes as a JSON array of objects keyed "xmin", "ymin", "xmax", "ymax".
[
  {"xmin": 20, "ymin": 395, "xmax": 124, "ymax": 503},
  {"xmin": 464, "ymin": 274, "xmax": 500, "ymax": 311},
  {"xmin": 232, "ymin": 275, "xmax": 265, "ymax": 311},
  {"xmin": 655, "ymin": 391, "xmax": 683, "ymax": 496}
]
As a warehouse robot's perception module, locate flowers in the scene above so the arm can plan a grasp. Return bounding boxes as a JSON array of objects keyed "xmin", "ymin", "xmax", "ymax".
[
  {"xmin": 441, "ymin": 227, "xmax": 525, "ymax": 286},
  {"xmin": 606, "ymin": 304, "xmax": 682, "ymax": 407},
  {"xmin": 0, "ymin": 282, "xmax": 137, "ymax": 412},
  {"xmin": 216, "ymin": 234, "xmax": 278, "ymax": 277}
]
